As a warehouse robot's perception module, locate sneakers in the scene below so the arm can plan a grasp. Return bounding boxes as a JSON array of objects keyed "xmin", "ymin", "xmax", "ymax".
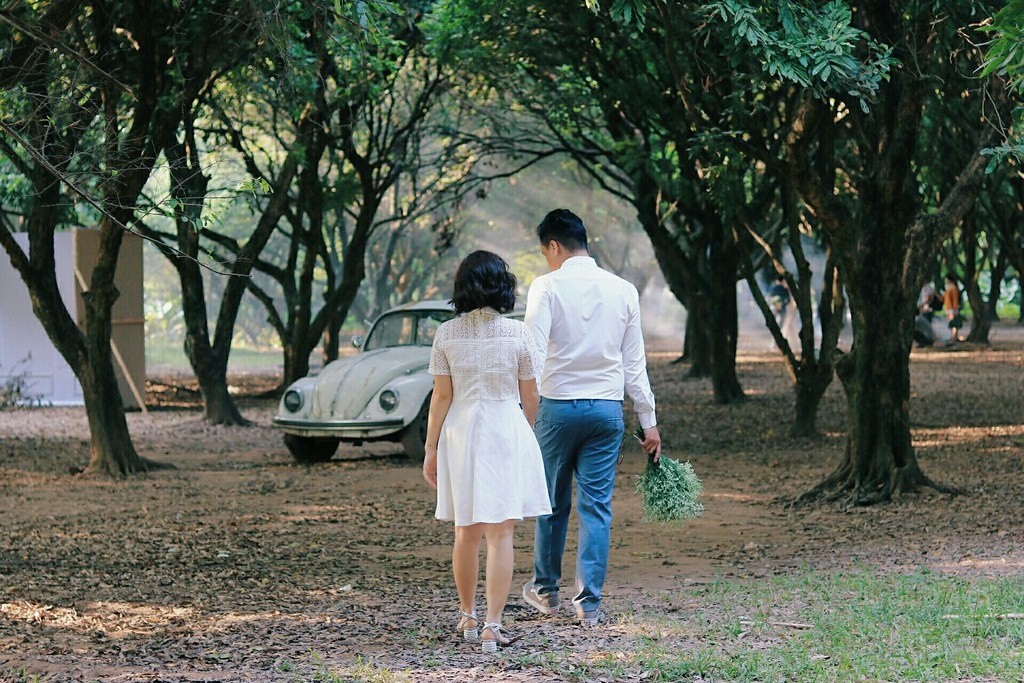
[
  {"xmin": 577, "ymin": 608, "xmax": 604, "ymax": 628},
  {"xmin": 522, "ymin": 582, "xmax": 559, "ymax": 614}
]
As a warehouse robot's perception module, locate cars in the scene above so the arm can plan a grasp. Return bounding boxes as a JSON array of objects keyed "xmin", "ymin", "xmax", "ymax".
[{"xmin": 268, "ymin": 298, "xmax": 527, "ymax": 464}]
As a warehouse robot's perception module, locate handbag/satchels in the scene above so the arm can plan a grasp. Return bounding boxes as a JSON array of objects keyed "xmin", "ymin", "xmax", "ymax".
[
  {"xmin": 949, "ymin": 314, "xmax": 963, "ymax": 330},
  {"xmin": 928, "ymin": 293, "xmax": 944, "ymax": 311}
]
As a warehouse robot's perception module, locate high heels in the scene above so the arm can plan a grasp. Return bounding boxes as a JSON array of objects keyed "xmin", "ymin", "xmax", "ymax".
[
  {"xmin": 456, "ymin": 610, "xmax": 480, "ymax": 641},
  {"xmin": 481, "ymin": 620, "xmax": 510, "ymax": 653}
]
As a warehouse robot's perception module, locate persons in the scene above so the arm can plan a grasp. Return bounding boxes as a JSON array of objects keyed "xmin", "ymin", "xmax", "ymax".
[
  {"xmin": 770, "ymin": 274, "xmax": 802, "ymax": 354},
  {"xmin": 423, "ymin": 250, "xmax": 552, "ymax": 656},
  {"xmin": 523, "ymin": 209, "xmax": 661, "ymax": 626},
  {"xmin": 914, "ymin": 273, "xmax": 967, "ymax": 348}
]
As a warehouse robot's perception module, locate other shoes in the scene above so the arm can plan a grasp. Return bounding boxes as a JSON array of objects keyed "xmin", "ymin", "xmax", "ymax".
[{"xmin": 946, "ymin": 342, "xmax": 955, "ymax": 347}]
